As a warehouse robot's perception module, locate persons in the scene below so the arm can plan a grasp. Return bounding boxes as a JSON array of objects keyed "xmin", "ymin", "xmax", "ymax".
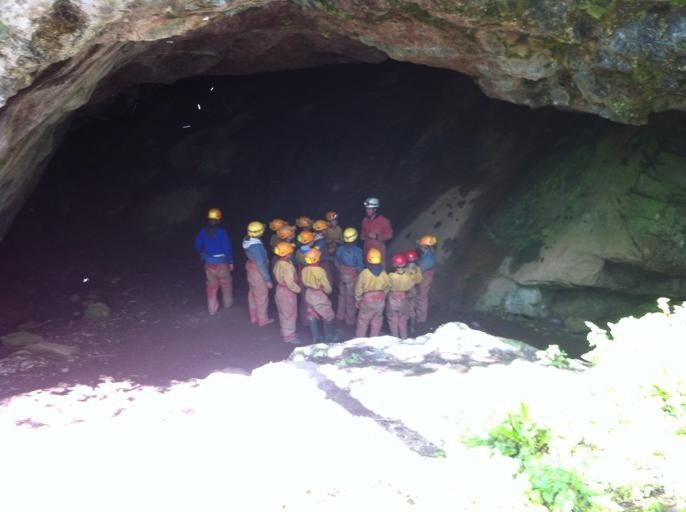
[
  {"xmin": 241, "ymin": 197, "xmax": 437, "ymax": 346},
  {"xmin": 194, "ymin": 207, "xmax": 238, "ymax": 316}
]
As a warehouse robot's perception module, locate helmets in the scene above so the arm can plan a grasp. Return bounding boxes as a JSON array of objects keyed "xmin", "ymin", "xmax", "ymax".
[
  {"xmin": 364, "ymin": 198, "xmax": 379, "ymax": 207},
  {"xmin": 391, "ymin": 250, "xmax": 418, "ymax": 267},
  {"xmin": 268, "ymin": 211, "xmax": 337, "ymax": 264},
  {"xmin": 343, "ymin": 227, "xmax": 358, "ymax": 243},
  {"xmin": 247, "ymin": 221, "xmax": 265, "ymax": 237},
  {"xmin": 208, "ymin": 208, "xmax": 222, "ymax": 220},
  {"xmin": 367, "ymin": 248, "xmax": 382, "ymax": 265},
  {"xmin": 418, "ymin": 235, "xmax": 436, "ymax": 245}
]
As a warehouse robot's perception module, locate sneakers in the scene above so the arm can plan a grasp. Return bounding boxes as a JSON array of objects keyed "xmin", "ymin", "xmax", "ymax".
[
  {"xmin": 289, "ymin": 338, "xmax": 301, "ymax": 345},
  {"xmin": 259, "ymin": 319, "xmax": 274, "ymax": 327}
]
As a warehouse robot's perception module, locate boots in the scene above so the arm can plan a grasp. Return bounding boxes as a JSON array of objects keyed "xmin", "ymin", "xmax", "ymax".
[
  {"xmin": 310, "ymin": 319, "xmax": 323, "ymax": 342},
  {"xmin": 408, "ymin": 318, "xmax": 417, "ymax": 335},
  {"xmin": 324, "ymin": 321, "xmax": 339, "ymax": 342}
]
